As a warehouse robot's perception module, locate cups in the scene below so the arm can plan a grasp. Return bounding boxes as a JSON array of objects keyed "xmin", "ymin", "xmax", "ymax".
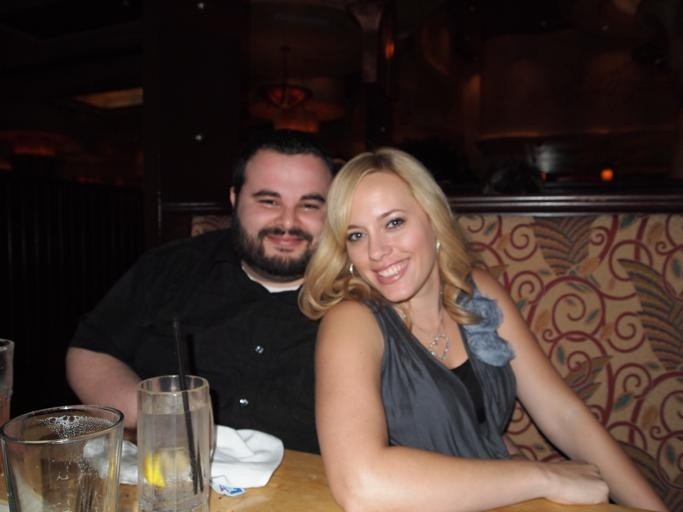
[
  {"xmin": 137, "ymin": 374, "xmax": 217, "ymax": 512},
  {"xmin": 0, "ymin": 404, "xmax": 126, "ymax": 511},
  {"xmin": 0, "ymin": 338, "xmax": 15, "ymax": 465}
]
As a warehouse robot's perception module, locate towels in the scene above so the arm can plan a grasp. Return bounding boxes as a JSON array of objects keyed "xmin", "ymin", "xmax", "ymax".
[{"xmin": 82, "ymin": 425, "xmax": 284, "ymax": 496}]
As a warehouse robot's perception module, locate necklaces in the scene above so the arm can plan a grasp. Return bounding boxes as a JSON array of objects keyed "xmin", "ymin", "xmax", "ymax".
[{"xmin": 400, "ymin": 285, "xmax": 451, "ymax": 361}]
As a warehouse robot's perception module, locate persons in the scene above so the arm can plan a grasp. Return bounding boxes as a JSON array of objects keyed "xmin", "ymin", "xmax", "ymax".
[
  {"xmin": 64, "ymin": 129, "xmax": 334, "ymax": 456},
  {"xmin": 246, "ymin": 76, "xmax": 349, "ymax": 132},
  {"xmin": 298, "ymin": 148, "xmax": 669, "ymax": 512}
]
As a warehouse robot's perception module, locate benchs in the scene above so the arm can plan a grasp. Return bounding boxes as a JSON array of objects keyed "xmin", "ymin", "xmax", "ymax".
[{"xmin": 153, "ymin": 191, "xmax": 683, "ymax": 512}]
